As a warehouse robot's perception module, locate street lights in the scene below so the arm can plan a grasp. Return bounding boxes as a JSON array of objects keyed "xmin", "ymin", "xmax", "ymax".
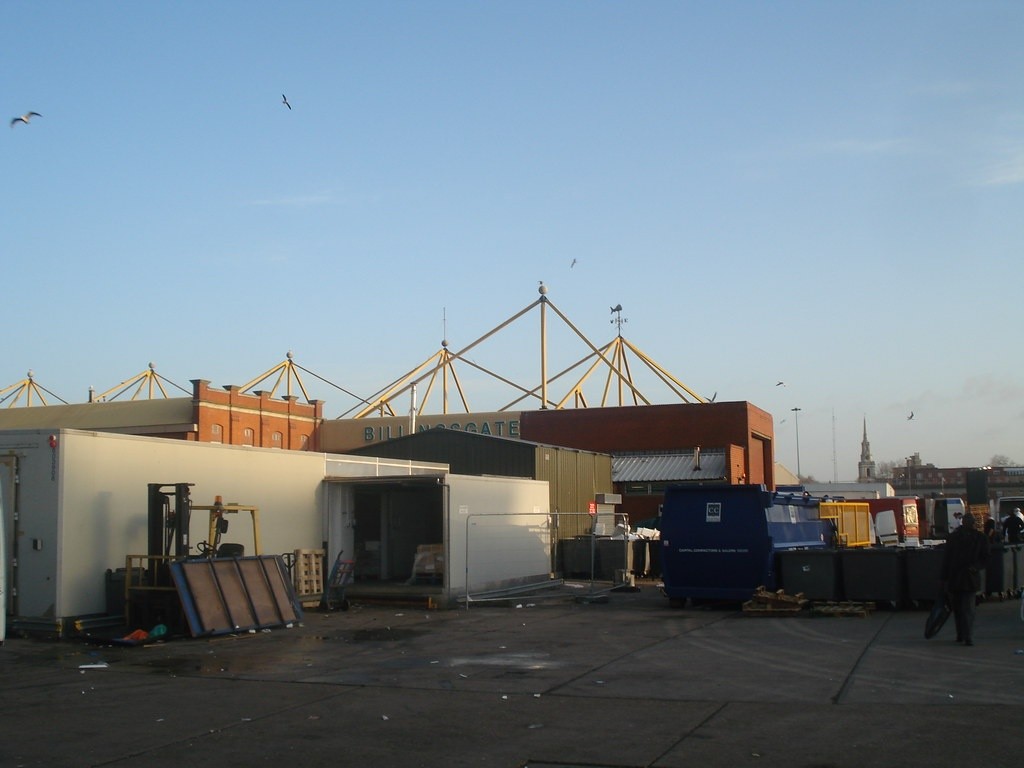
[{"xmin": 790, "ymin": 408, "xmax": 802, "ymax": 480}]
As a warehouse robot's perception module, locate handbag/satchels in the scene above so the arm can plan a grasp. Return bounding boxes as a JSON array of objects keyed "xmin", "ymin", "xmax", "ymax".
[{"xmin": 924, "ymin": 602, "xmax": 952, "ymax": 639}]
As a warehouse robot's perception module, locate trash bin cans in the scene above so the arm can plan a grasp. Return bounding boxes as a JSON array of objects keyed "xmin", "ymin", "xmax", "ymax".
[
  {"xmin": 560, "ymin": 534, "xmax": 660, "ymax": 582},
  {"xmin": 776, "ymin": 543, "xmax": 1024, "ymax": 611}
]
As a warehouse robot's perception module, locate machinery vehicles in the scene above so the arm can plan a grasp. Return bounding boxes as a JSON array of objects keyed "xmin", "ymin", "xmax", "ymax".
[{"xmin": 69, "ymin": 482, "xmax": 262, "ymax": 645}]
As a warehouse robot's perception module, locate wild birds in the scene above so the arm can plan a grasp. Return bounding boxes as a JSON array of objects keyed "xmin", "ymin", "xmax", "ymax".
[
  {"xmin": 776, "ymin": 381, "xmax": 785, "ymax": 387},
  {"xmin": 282, "ymin": 95, "xmax": 291, "ymax": 110},
  {"xmin": 907, "ymin": 411, "xmax": 915, "ymax": 421},
  {"xmin": 10, "ymin": 111, "xmax": 42, "ymax": 127},
  {"xmin": 570, "ymin": 259, "xmax": 576, "ymax": 268}
]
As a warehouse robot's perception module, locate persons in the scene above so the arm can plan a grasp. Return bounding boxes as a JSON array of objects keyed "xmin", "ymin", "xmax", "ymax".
[
  {"xmin": 940, "ymin": 512, "xmax": 990, "ymax": 645},
  {"xmin": 984, "ymin": 507, "xmax": 1024, "ymax": 544}
]
